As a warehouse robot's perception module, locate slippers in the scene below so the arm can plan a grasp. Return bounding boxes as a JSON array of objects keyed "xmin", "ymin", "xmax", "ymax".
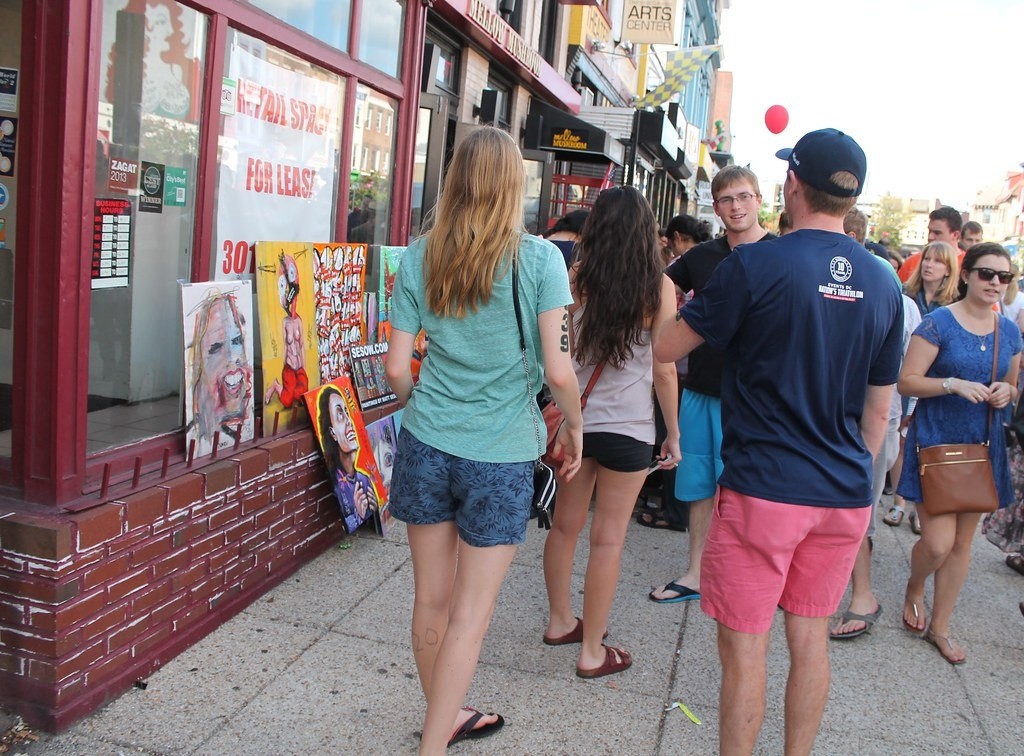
[
  {"xmin": 909, "ymin": 511, "xmax": 921, "ymax": 533},
  {"xmin": 637, "ymin": 512, "xmax": 686, "ymax": 531},
  {"xmin": 829, "ymin": 604, "xmax": 883, "ymax": 638},
  {"xmin": 543, "ymin": 617, "xmax": 608, "ymax": 644},
  {"xmin": 577, "ymin": 644, "xmax": 632, "ymax": 677},
  {"xmin": 923, "ymin": 634, "xmax": 965, "ymax": 663},
  {"xmin": 447, "ymin": 707, "xmax": 504, "ymax": 746},
  {"xmin": 649, "ymin": 580, "xmax": 701, "ymax": 602},
  {"xmin": 884, "ymin": 506, "xmax": 904, "ymax": 525},
  {"xmin": 903, "ymin": 579, "xmax": 926, "ymax": 632}
]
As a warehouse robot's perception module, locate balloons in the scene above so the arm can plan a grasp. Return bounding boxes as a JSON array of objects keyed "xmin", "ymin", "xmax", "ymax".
[{"xmin": 765, "ymin": 104, "xmax": 789, "ymax": 135}]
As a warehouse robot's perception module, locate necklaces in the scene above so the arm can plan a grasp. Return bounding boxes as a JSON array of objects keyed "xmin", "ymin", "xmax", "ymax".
[{"xmin": 975, "ymin": 327, "xmax": 986, "ymax": 351}]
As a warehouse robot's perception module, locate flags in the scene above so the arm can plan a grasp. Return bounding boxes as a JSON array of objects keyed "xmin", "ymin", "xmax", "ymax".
[{"xmin": 635, "ymin": 44, "xmax": 721, "ymax": 108}]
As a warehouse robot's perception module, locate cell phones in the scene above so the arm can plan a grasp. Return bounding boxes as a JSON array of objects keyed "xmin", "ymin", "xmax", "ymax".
[{"xmin": 647, "ymin": 453, "xmax": 671, "ymax": 475}]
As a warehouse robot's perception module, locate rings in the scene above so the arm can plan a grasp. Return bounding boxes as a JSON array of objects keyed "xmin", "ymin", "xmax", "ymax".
[{"xmin": 674, "ymin": 464, "xmax": 678, "ymax": 466}]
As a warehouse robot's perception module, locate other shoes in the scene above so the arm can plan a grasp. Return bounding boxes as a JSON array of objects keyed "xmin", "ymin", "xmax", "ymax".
[{"xmin": 1006, "ymin": 554, "xmax": 1024, "ymax": 575}]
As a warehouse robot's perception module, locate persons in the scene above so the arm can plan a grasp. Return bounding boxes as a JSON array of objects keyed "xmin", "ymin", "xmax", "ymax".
[
  {"xmin": 532, "ymin": 128, "xmax": 1024, "ymax": 756},
  {"xmin": 388, "ymin": 125, "xmax": 584, "ymax": 756}
]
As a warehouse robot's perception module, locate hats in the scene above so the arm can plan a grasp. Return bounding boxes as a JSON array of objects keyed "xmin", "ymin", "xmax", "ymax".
[
  {"xmin": 776, "ymin": 128, "xmax": 866, "ymax": 197},
  {"xmin": 867, "ymin": 243, "xmax": 890, "ymax": 261}
]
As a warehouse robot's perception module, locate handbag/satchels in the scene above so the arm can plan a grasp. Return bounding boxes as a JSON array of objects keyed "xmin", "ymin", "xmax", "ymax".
[
  {"xmin": 530, "ymin": 460, "xmax": 557, "ymax": 530},
  {"xmin": 917, "ymin": 443, "xmax": 999, "ymax": 515},
  {"xmin": 542, "ymin": 403, "xmax": 565, "ymax": 467}
]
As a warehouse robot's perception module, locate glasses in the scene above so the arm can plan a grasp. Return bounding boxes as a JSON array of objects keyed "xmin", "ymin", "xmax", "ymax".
[
  {"xmin": 716, "ymin": 194, "xmax": 756, "ymax": 207},
  {"xmin": 970, "ymin": 268, "xmax": 1015, "ymax": 284}
]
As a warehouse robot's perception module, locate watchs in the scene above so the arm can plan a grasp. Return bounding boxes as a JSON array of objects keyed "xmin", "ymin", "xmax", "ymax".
[{"xmin": 943, "ymin": 377, "xmax": 954, "ymax": 395}]
